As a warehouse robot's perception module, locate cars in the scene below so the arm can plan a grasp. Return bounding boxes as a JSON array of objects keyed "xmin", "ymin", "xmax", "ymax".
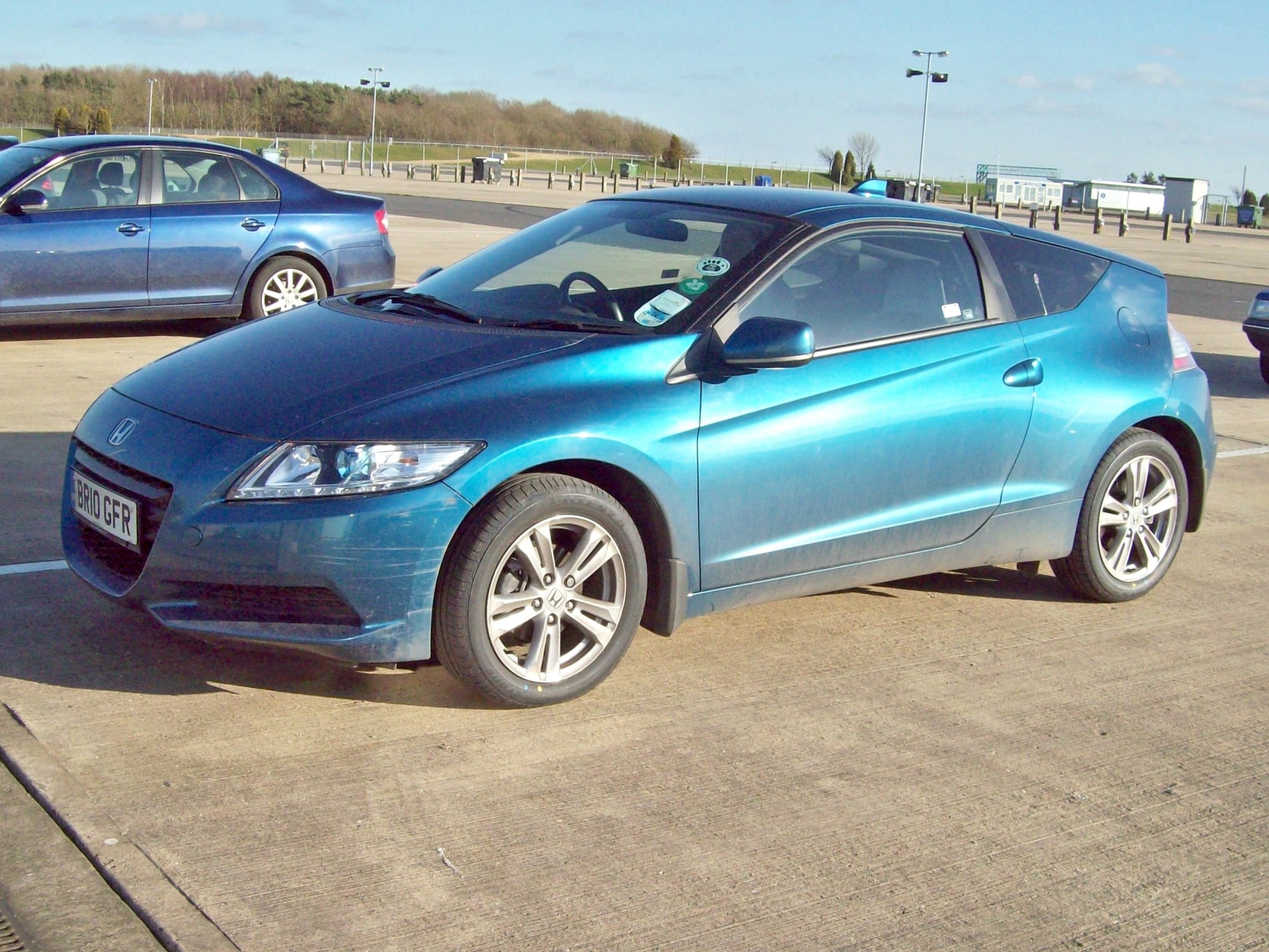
[
  {"xmin": 0, "ymin": 134, "xmax": 395, "ymax": 322},
  {"xmin": 1241, "ymin": 289, "xmax": 1269, "ymax": 386},
  {"xmin": 0, "ymin": 135, "xmax": 55, "ymax": 198},
  {"xmin": 274, "ymin": 141, "xmax": 289, "ymax": 158}
]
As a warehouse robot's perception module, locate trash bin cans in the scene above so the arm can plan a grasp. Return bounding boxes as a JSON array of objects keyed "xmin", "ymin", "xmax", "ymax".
[
  {"xmin": 470, "ymin": 156, "xmax": 505, "ymax": 184},
  {"xmin": 755, "ymin": 176, "xmax": 772, "ymax": 187},
  {"xmin": 927, "ymin": 184, "xmax": 941, "ymax": 202},
  {"xmin": 1234, "ymin": 204, "xmax": 1264, "ymax": 228},
  {"xmin": 620, "ymin": 163, "xmax": 638, "ymax": 180},
  {"xmin": 256, "ymin": 148, "xmax": 282, "ymax": 165},
  {"xmin": 887, "ymin": 178, "xmax": 928, "ymax": 202}
]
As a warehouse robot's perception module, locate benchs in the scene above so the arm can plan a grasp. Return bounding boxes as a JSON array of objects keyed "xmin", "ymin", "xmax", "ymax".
[{"xmin": 206, "ymin": 162, "xmax": 265, "ymax": 199}]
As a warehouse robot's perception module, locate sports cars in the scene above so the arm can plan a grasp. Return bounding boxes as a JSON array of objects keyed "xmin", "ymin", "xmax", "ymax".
[{"xmin": 61, "ymin": 187, "xmax": 1216, "ymax": 711}]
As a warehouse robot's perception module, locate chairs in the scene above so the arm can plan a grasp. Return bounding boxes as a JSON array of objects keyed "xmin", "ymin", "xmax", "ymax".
[
  {"xmin": 107, "ymin": 171, "xmax": 135, "ymax": 206},
  {"xmin": 98, "ymin": 162, "xmax": 124, "ymax": 203},
  {"xmin": 838, "ymin": 256, "xmax": 942, "ymax": 341},
  {"xmin": 719, "ymin": 222, "xmax": 797, "ymax": 322}
]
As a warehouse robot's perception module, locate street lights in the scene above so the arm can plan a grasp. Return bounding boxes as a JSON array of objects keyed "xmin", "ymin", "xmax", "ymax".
[
  {"xmin": 906, "ymin": 50, "xmax": 949, "ymax": 203},
  {"xmin": 360, "ymin": 67, "xmax": 391, "ymax": 176},
  {"xmin": 148, "ymin": 77, "xmax": 158, "ymax": 135}
]
